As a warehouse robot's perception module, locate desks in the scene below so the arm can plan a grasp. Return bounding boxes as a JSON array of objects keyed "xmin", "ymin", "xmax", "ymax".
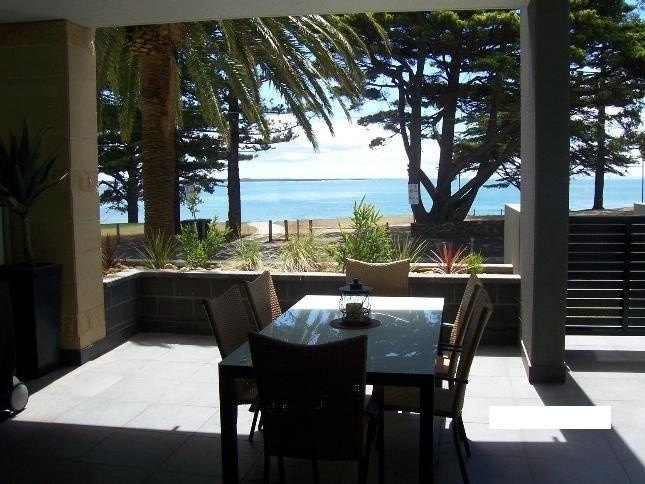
[{"xmin": 219, "ymin": 292, "xmax": 449, "ymax": 484}]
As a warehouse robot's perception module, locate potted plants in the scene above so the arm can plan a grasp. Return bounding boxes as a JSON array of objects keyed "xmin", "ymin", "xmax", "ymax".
[
  {"xmin": 0, "ymin": 118, "xmax": 72, "ymax": 378},
  {"xmin": 179, "ymin": 184, "xmax": 211, "ymax": 240}
]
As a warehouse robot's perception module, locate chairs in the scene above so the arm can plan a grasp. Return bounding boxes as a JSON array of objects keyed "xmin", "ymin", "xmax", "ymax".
[
  {"xmin": 248, "ymin": 330, "xmax": 387, "ymax": 484},
  {"xmin": 242, "ymin": 270, "xmax": 283, "ymax": 442},
  {"xmin": 369, "ymin": 272, "xmax": 484, "ymax": 442},
  {"xmin": 366, "ymin": 289, "xmax": 496, "ymax": 483},
  {"xmin": 201, "ymin": 280, "xmax": 323, "ymax": 453},
  {"xmin": 344, "ymin": 256, "xmax": 411, "ymax": 296}
]
